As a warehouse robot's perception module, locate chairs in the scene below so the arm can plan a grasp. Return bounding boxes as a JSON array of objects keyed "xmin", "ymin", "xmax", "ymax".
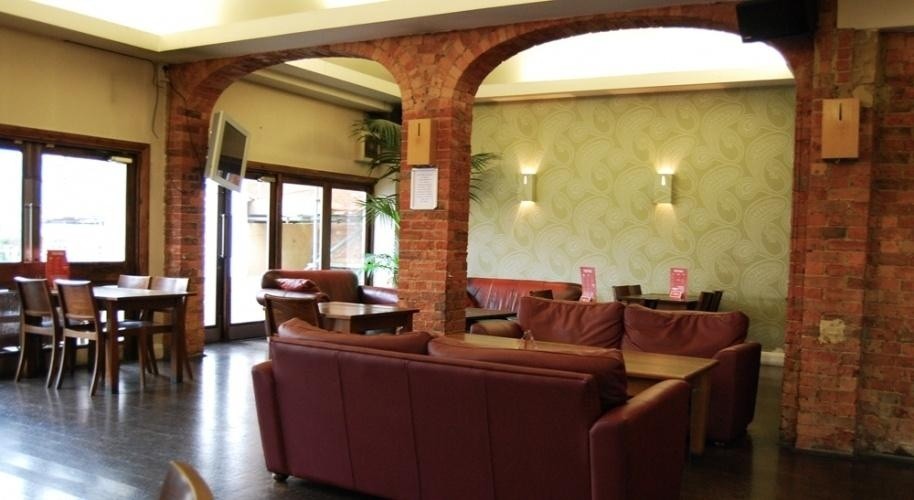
[{"xmin": 13, "ymin": 275, "xmax": 199, "ymax": 396}]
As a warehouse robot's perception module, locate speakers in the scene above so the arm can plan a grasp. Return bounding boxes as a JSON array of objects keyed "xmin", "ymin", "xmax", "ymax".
[
  {"xmin": 821, "ymin": 98, "xmax": 860, "ymax": 160},
  {"xmin": 407, "ymin": 119, "xmax": 437, "ymax": 166},
  {"xmin": 735, "ymin": 0, "xmax": 818, "ymax": 42}
]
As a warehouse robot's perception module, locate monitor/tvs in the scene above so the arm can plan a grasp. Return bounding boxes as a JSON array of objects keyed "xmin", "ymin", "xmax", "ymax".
[{"xmin": 202, "ymin": 111, "xmax": 251, "ymax": 195}]
